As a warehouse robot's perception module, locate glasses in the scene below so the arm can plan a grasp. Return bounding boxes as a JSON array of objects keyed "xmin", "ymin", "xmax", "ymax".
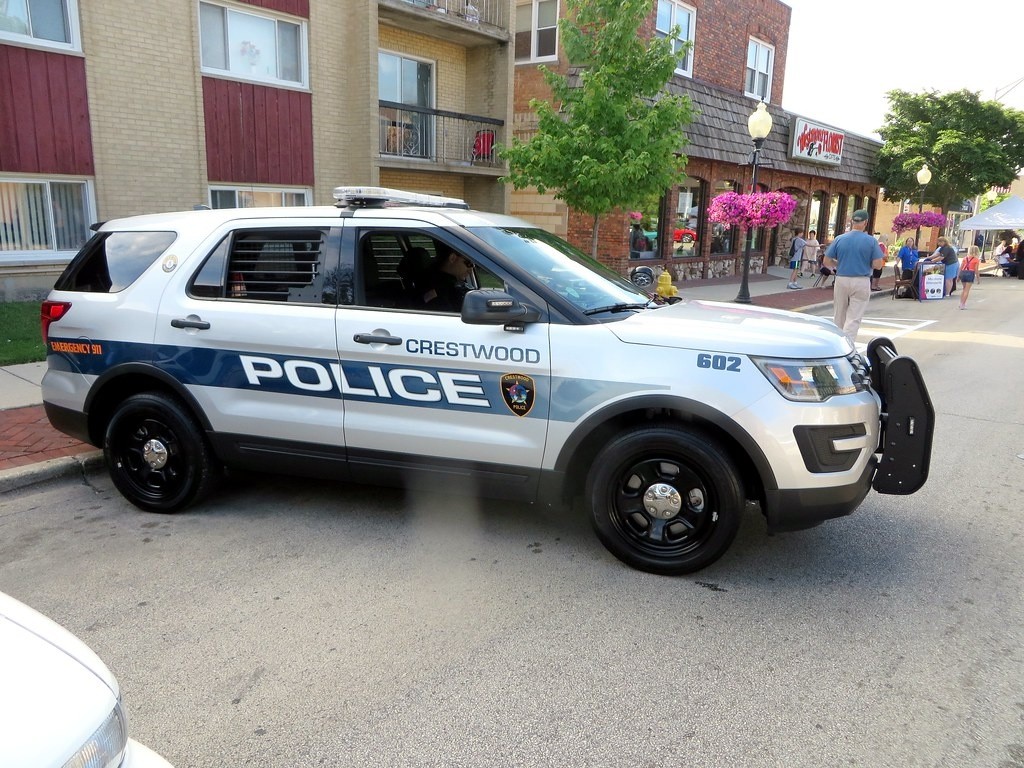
[{"xmin": 463, "ymin": 260, "xmax": 474, "ymax": 268}]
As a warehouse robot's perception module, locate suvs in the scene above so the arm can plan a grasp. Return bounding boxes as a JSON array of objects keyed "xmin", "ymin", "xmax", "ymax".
[{"xmin": 38, "ymin": 185, "xmax": 937, "ymax": 577}]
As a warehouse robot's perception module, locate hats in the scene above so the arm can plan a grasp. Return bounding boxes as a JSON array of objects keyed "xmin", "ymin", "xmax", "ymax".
[{"xmin": 852, "ymin": 209, "xmax": 868, "ymax": 222}]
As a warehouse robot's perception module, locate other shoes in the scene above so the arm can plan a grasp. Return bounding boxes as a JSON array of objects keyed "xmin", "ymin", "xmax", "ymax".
[
  {"xmin": 809, "ymin": 274, "xmax": 815, "ymax": 279},
  {"xmin": 797, "ymin": 272, "xmax": 804, "ymax": 279},
  {"xmin": 787, "ymin": 284, "xmax": 803, "ymax": 289}
]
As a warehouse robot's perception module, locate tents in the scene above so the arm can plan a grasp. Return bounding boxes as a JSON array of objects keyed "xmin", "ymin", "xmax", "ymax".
[{"xmin": 958, "ymin": 194, "xmax": 1024, "ymax": 259}]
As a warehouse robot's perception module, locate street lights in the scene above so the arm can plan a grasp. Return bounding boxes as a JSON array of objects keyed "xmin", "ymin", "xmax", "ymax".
[
  {"xmin": 978, "ymin": 190, "xmax": 998, "ymax": 263},
  {"xmin": 733, "ymin": 96, "xmax": 773, "ymax": 303},
  {"xmin": 915, "ymin": 164, "xmax": 932, "ymax": 247}
]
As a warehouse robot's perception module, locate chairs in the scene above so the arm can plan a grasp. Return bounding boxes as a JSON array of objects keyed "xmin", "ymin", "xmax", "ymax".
[
  {"xmin": 994, "ymin": 257, "xmax": 1009, "ymax": 277},
  {"xmin": 470, "ymin": 129, "xmax": 497, "ymax": 168},
  {"xmin": 891, "ymin": 262, "xmax": 921, "ymax": 301},
  {"xmin": 397, "ymin": 246, "xmax": 432, "ymax": 310}
]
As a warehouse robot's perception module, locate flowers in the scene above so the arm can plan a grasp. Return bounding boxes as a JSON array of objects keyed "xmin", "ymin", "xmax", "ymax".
[
  {"xmin": 707, "ymin": 191, "xmax": 799, "ymax": 231},
  {"xmin": 891, "ymin": 211, "xmax": 947, "ymax": 236}
]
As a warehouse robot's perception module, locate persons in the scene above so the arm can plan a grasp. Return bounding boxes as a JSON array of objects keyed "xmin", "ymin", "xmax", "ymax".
[
  {"xmin": 786, "ymin": 228, "xmax": 825, "ymax": 289},
  {"xmin": 925, "ymin": 237, "xmax": 959, "ymax": 299},
  {"xmin": 797, "ymin": 230, "xmax": 820, "ymax": 279},
  {"xmin": 424, "ymin": 238, "xmax": 475, "ymax": 315},
  {"xmin": 629, "ymin": 224, "xmax": 644, "ymax": 258},
  {"xmin": 821, "ymin": 262, "xmax": 837, "ymax": 289},
  {"xmin": 976, "ymin": 235, "xmax": 1024, "ymax": 279},
  {"xmin": 957, "ymin": 246, "xmax": 980, "ymax": 310},
  {"xmin": 896, "ymin": 237, "xmax": 918, "ymax": 280},
  {"xmin": 823, "ymin": 209, "xmax": 884, "ymax": 345},
  {"xmin": 870, "ymin": 234, "xmax": 888, "ymax": 291}
]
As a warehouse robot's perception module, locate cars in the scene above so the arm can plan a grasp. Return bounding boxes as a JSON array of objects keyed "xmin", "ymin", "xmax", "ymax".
[
  {"xmin": 0, "ymin": 589, "xmax": 183, "ymax": 768},
  {"xmin": 674, "ymin": 227, "xmax": 697, "ymax": 243},
  {"xmin": 630, "ymin": 228, "xmax": 657, "ymax": 239}
]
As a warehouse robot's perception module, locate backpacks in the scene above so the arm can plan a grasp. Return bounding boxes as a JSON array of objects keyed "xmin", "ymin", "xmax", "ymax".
[{"xmin": 789, "ymin": 237, "xmax": 801, "ymax": 257}]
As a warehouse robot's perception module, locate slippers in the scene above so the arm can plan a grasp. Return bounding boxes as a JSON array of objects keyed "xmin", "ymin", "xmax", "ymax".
[{"xmin": 871, "ymin": 287, "xmax": 882, "ymax": 291}]
